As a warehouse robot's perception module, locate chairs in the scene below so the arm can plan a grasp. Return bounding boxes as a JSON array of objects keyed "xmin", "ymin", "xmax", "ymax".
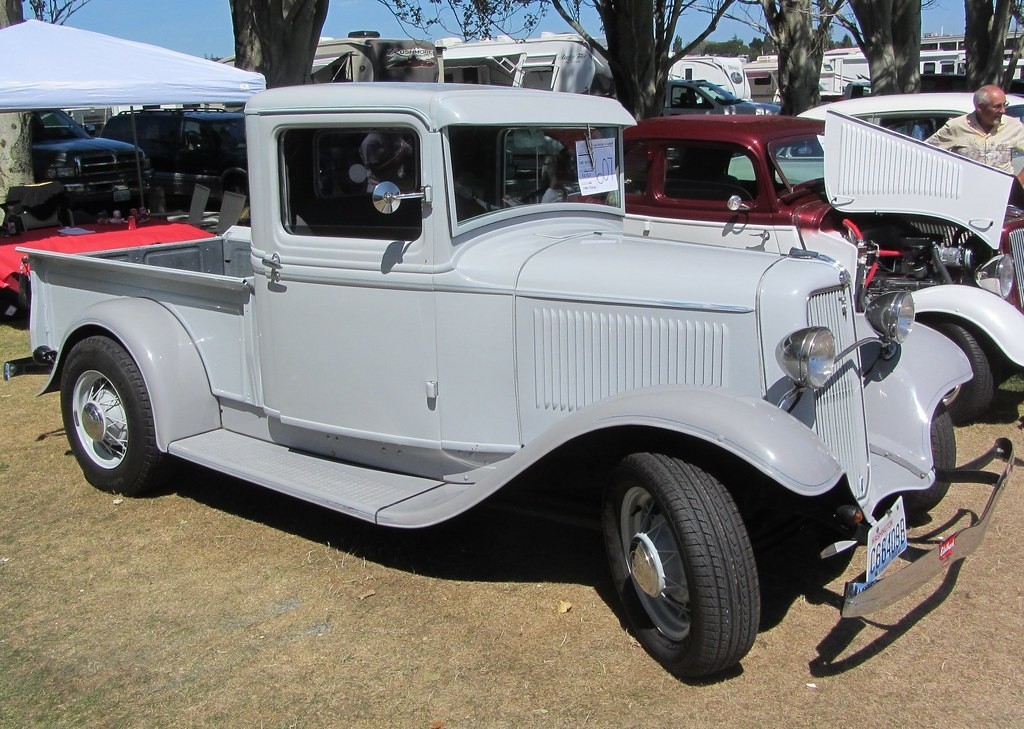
[
  {"xmin": 204, "ymin": 191, "xmax": 246, "ymax": 237},
  {"xmin": 177, "ymin": 184, "xmax": 210, "ymax": 228}
]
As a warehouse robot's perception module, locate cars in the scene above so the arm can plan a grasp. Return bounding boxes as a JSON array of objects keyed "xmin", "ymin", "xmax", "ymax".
[
  {"xmin": 728, "ymin": 92, "xmax": 1023, "ymax": 187},
  {"xmin": 30, "ymin": 109, "xmax": 153, "ymax": 226}
]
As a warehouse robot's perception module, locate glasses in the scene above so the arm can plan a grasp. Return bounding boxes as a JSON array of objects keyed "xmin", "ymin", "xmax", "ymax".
[{"xmin": 982, "ymin": 101, "xmax": 1009, "ymax": 111}]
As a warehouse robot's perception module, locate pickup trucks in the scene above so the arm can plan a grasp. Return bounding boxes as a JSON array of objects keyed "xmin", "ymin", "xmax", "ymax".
[
  {"xmin": 2, "ymin": 80, "xmax": 1016, "ymax": 680},
  {"xmin": 663, "ymin": 79, "xmax": 781, "ymax": 114},
  {"xmin": 543, "ymin": 113, "xmax": 1024, "ymax": 313}
]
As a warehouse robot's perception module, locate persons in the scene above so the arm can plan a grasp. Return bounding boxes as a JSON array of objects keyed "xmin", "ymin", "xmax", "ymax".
[
  {"xmin": 359, "ymin": 127, "xmax": 412, "ymax": 194},
  {"xmin": 923, "ymin": 85, "xmax": 1024, "ymax": 211}
]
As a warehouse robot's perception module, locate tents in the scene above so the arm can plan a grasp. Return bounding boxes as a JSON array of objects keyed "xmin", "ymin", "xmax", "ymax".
[{"xmin": 0, "ymin": 19, "xmax": 267, "ymax": 214}]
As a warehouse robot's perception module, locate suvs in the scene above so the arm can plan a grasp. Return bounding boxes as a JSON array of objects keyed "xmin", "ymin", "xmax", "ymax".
[{"xmin": 100, "ymin": 109, "xmax": 249, "ymax": 205}]
